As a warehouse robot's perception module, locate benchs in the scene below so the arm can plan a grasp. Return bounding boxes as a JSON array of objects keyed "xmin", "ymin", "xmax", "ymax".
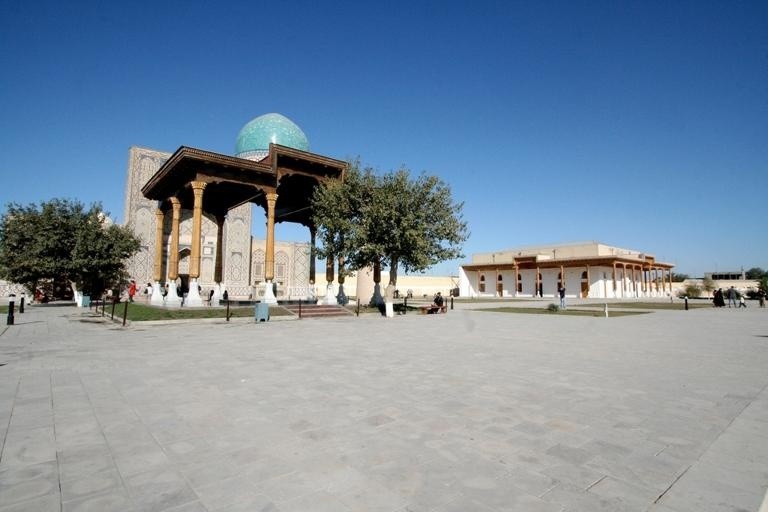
[{"xmin": 419, "ymin": 306, "xmax": 447, "ymax": 315}]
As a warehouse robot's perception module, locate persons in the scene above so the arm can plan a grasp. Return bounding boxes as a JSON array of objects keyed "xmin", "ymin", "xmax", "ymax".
[
  {"xmin": 127, "ymin": 279, "xmax": 136, "ymax": 303},
  {"xmin": 726, "ymin": 285, "xmax": 737, "ymax": 308},
  {"xmin": 738, "ymin": 294, "xmax": 747, "ymax": 308},
  {"xmin": 430, "ymin": 291, "xmax": 443, "ymax": 307},
  {"xmin": 713, "ymin": 288, "xmax": 722, "ymax": 308},
  {"xmin": 756, "ymin": 288, "xmax": 767, "ymax": 308},
  {"xmin": 35, "ymin": 287, "xmax": 40, "ymax": 304},
  {"xmin": 557, "ymin": 285, "xmax": 566, "ymax": 309}
]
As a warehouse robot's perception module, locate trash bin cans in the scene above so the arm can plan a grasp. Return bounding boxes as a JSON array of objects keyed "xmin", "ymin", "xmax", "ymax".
[
  {"xmin": 254, "ymin": 303, "xmax": 269, "ymax": 322},
  {"xmin": 83, "ymin": 296, "xmax": 90, "ymax": 307}
]
